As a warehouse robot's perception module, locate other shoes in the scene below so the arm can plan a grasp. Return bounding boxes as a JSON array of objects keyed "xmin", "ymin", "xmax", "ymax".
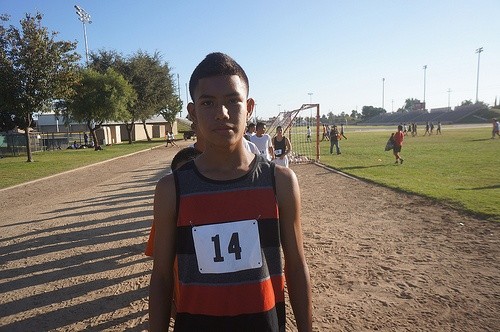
[
  {"xmin": 395, "ymin": 161, "xmax": 398, "ymax": 163},
  {"xmin": 401, "ymin": 159, "xmax": 403, "ymax": 164}
]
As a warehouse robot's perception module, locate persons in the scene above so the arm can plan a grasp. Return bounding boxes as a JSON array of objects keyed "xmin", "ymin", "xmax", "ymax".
[
  {"xmin": 392, "ymin": 125, "xmax": 404, "ymax": 165},
  {"xmin": 492, "ymin": 120, "xmax": 500, "ymax": 139},
  {"xmin": 306, "ymin": 126, "xmax": 311, "ymax": 142},
  {"xmin": 321, "ymin": 123, "xmax": 347, "ymax": 142},
  {"xmin": 149, "ymin": 52, "xmax": 312, "ymax": 332},
  {"xmin": 144, "ymin": 123, "xmax": 292, "ymax": 257},
  {"xmin": 74, "ymin": 141, "xmax": 79, "ymax": 148},
  {"xmin": 422, "ymin": 121, "xmax": 442, "ymax": 136},
  {"xmin": 330, "ymin": 124, "xmax": 341, "ymax": 155},
  {"xmin": 84, "ymin": 134, "xmax": 88, "ymax": 145},
  {"xmin": 399, "ymin": 122, "xmax": 417, "ymax": 137}
]
